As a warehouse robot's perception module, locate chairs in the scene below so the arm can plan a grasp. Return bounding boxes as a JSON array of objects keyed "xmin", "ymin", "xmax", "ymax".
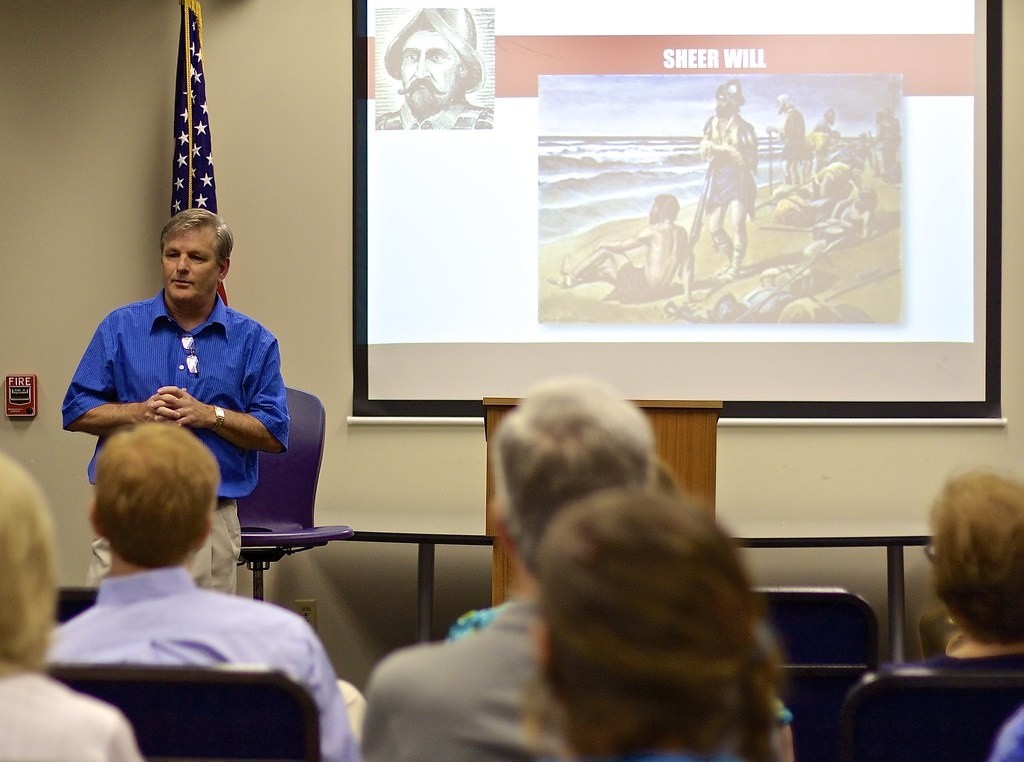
[
  {"xmin": 235, "ymin": 387, "xmax": 355, "ymax": 602},
  {"xmin": 836, "ymin": 664, "xmax": 1024, "ymax": 762},
  {"xmin": 748, "ymin": 586, "xmax": 881, "ymax": 762},
  {"xmin": 40, "ymin": 664, "xmax": 321, "ymax": 762}
]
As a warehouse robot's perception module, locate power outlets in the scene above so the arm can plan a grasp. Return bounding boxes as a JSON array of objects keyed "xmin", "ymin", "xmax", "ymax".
[{"xmin": 294, "ymin": 599, "xmax": 317, "ymax": 631}]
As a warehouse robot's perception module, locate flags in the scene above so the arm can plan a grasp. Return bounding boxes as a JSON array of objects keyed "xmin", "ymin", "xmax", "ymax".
[{"xmin": 170, "ymin": 0, "xmax": 228, "ymax": 308}]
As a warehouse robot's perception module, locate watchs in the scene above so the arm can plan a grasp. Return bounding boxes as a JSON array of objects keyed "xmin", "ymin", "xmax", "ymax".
[{"xmin": 210, "ymin": 405, "xmax": 225, "ymax": 432}]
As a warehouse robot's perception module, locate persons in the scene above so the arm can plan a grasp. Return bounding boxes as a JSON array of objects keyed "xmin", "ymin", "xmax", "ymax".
[
  {"xmin": 47, "ymin": 422, "xmax": 368, "ymax": 762},
  {"xmin": 61, "ymin": 209, "xmax": 290, "ymax": 597},
  {"xmin": 834, "ymin": 470, "xmax": 1024, "ymax": 709},
  {"xmin": 0, "ymin": 452, "xmax": 145, "ymax": 762},
  {"xmin": 363, "ymin": 378, "xmax": 793, "ymax": 762}
]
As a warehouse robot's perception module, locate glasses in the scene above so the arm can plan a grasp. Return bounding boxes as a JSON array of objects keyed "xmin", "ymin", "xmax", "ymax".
[{"xmin": 181, "ymin": 331, "xmax": 200, "ymax": 374}]
